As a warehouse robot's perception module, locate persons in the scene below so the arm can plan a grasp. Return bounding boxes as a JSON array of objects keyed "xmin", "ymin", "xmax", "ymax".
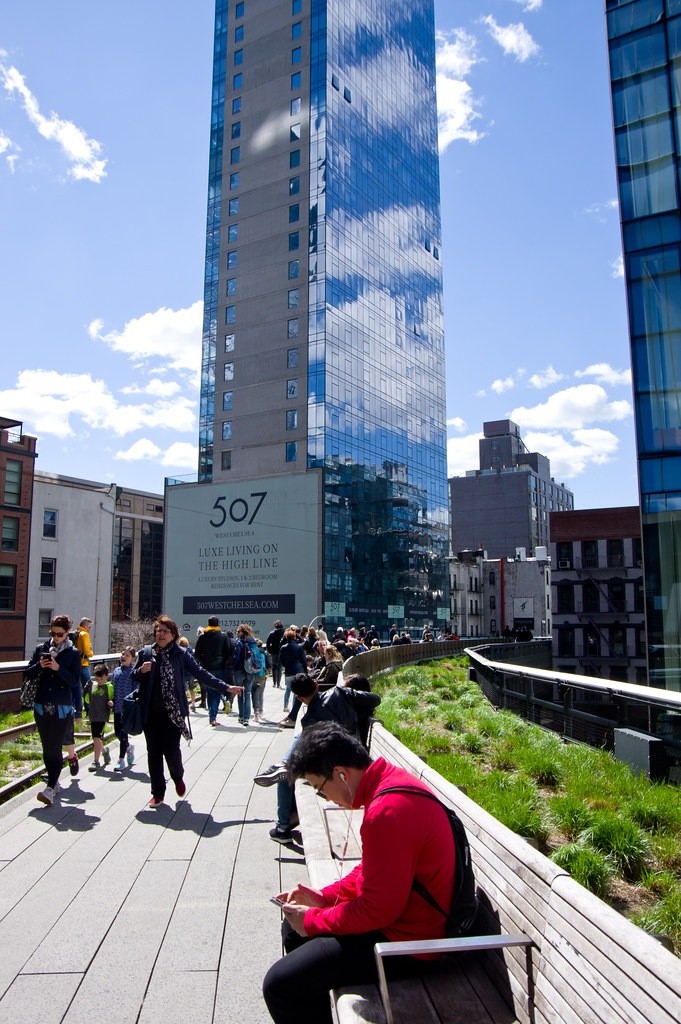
[
  {"xmin": 420, "ymin": 624, "xmax": 435, "ymax": 643},
  {"xmin": 265, "ymin": 620, "xmax": 344, "ymax": 728},
  {"xmin": 331, "ymin": 622, "xmax": 382, "ymax": 661},
  {"xmin": 435, "ymin": 590, "xmax": 442, "ymax": 601},
  {"xmin": 194, "ymin": 616, "xmax": 236, "ymax": 726},
  {"xmin": 67, "ymin": 616, "xmax": 98, "ymax": 718},
  {"xmin": 111, "ymin": 646, "xmax": 137, "ymax": 771},
  {"xmin": 233, "ymin": 624, "xmax": 273, "ymax": 726},
  {"xmin": 439, "ymin": 608, "xmax": 448, "ymax": 619},
  {"xmin": 389, "ymin": 624, "xmax": 412, "ymax": 646},
  {"xmin": 501, "ymin": 624, "xmax": 534, "ymax": 642},
  {"xmin": 20, "ymin": 615, "xmax": 83, "ymax": 805},
  {"xmin": 263, "ymin": 721, "xmax": 476, "ymax": 1024},
  {"xmin": 254, "ymin": 673, "xmax": 382, "ymax": 844},
  {"xmin": 85, "ymin": 664, "xmax": 115, "ymax": 770},
  {"xmin": 438, "ymin": 626, "xmax": 460, "ymax": 641},
  {"xmin": 178, "ymin": 636, "xmax": 196, "ymax": 712},
  {"xmin": 122, "ymin": 615, "xmax": 245, "ymax": 807},
  {"xmin": 330, "ymin": 602, "xmax": 340, "ymax": 616},
  {"xmin": 391, "ymin": 605, "xmax": 401, "ymax": 618}
]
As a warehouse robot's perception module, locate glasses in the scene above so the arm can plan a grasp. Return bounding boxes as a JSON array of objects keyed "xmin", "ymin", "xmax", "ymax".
[
  {"xmin": 155, "ymin": 628, "xmax": 172, "ymax": 633},
  {"xmin": 236, "ymin": 631, "xmax": 243, "ymax": 633},
  {"xmin": 316, "ymin": 772, "xmax": 332, "ymax": 799},
  {"xmin": 49, "ymin": 629, "xmax": 69, "ymax": 637}
]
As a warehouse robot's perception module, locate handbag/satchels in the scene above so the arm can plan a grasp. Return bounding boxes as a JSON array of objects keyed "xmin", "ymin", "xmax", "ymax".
[
  {"xmin": 121, "ymin": 688, "xmax": 143, "ymax": 735},
  {"xmin": 20, "ymin": 678, "xmax": 39, "ymax": 707}
]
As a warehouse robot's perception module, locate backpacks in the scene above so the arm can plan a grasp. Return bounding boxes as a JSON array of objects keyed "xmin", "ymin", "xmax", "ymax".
[
  {"xmin": 67, "ymin": 630, "xmax": 86, "ymax": 648},
  {"xmin": 242, "ymin": 637, "xmax": 262, "ymax": 674},
  {"xmin": 367, "ymin": 785, "xmax": 476, "ymax": 938}
]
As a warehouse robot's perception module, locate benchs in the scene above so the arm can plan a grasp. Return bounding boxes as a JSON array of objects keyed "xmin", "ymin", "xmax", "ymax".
[{"xmin": 293, "ymin": 682, "xmax": 681, "ymax": 1024}]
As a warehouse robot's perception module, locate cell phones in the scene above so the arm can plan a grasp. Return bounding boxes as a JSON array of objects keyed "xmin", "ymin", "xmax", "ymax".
[
  {"xmin": 42, "ymin": 653, "xmax": 51, "ymax": 665},
  {"xmin": 270, "ymin": 897, "xmax": 285, "ymax": 906}
]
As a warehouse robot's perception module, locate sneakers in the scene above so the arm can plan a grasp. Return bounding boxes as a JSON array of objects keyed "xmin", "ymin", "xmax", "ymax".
[
  {"xmin": 114, "ymin": 760, "xmax": 125, "ymax": 770},
  {"xmin": 88, "ymin": 761, "xmax": 100, "ymax": 771},
  {"xmin": 102, "ymin": 747, "xmax": 111, "ymax": 764},
  {"xmin": 253, "ymin": 765, "xmax": 288, "ymax": 787},
  {"xmin": 40, "ymin": 773, "xmax": 49, "ymax": 782},
  {"xmin": 269, "ymin": 828, "xmax": 292, "ymax": 844},
  {"xmin": 68, "ymin": 752, "xmax": 80, "ymax": 776},
  {"xmin": 148, "ymin": 797, "xmax": 163, "ymax": 807},
  {"xmin": 54, "ymin": 781, "xmax": 60, "ymax": 794},
  {"xmin": 175, "ymin": 779, "xmax": 186, "ymax": 797},
  {"xmin": 37, "ymin": 787, "xmax": 54, "ymax": 805},
  {"xmin": 126, "ymin": 745, "xmax": 135, "ymax": 764}
]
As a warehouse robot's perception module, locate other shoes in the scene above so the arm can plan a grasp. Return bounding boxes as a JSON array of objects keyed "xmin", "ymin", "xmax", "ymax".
[
  {"xmin": 210, "ymin": 721, "xmax": 221, "ymax": 726},
  {"xmin": 191, "ymin": 706, "xmax": 195, "ymax": 712},
  {"xmin": 198, "ymin": 702, "xmax": 206, "ymax": 708},
  {"xmin": 272, "ymin": 683, "xmax": 276, "ymax": 687},
  {"xmin": 238, "ymin": 707, "xmax": 297, "ymax": 727},
  {"xmin": 225, "ymin": 701, "xmax": 230, "ymax": 714}
]
